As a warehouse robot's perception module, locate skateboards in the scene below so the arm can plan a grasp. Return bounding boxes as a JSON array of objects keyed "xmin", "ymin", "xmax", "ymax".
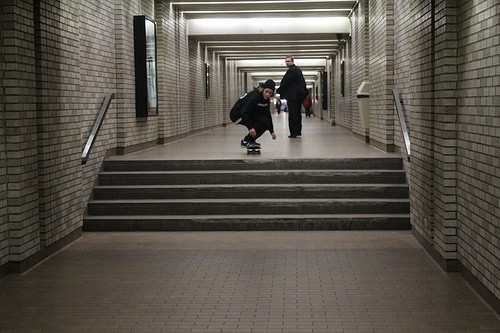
[{"xmin": 246, "ymin": 142, "xmax": 262, "ymax": 155}]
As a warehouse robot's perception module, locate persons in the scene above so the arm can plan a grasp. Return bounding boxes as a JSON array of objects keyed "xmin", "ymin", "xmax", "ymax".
[
  {"xmin": 272, "ymin": 56, "xmax": 309, "ymax": 138},
  {"xmin": 230, "ymin": 78, "xmax": 276, "ymax": 148},
  {"xmin": 276, "ymin": 98, "xmax": 282, "ymax": 114},
  {"xmin": 303, "ymin": 89, "xmax": 312, "ymax": 117}
]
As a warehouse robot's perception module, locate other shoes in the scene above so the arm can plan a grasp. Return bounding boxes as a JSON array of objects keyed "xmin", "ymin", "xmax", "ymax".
[
  {"xmin": 297, "ymin": 132, "xmax": 302, "ymax": 136},
  {"xmin": 253, "ymin": 141, "xmax": 261, "ymax": 146},
  {"xmin": 241, "ymin": 140, "xmax": 260, "ymax": 147},
  {"xmin": 288, "ymin": 132, "xmax": 296, "ymax": 138}
]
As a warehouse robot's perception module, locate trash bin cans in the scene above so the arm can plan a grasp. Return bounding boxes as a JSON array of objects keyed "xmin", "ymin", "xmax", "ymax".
[{"xmin": 356, "ymin": 82, "xmax": 370, "ymax": 142}]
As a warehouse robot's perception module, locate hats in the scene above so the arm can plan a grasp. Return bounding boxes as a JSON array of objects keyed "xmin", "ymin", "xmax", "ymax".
[{"xmin": 261, "ymin": 78, "xmax": 276, "ymax": 91}]
